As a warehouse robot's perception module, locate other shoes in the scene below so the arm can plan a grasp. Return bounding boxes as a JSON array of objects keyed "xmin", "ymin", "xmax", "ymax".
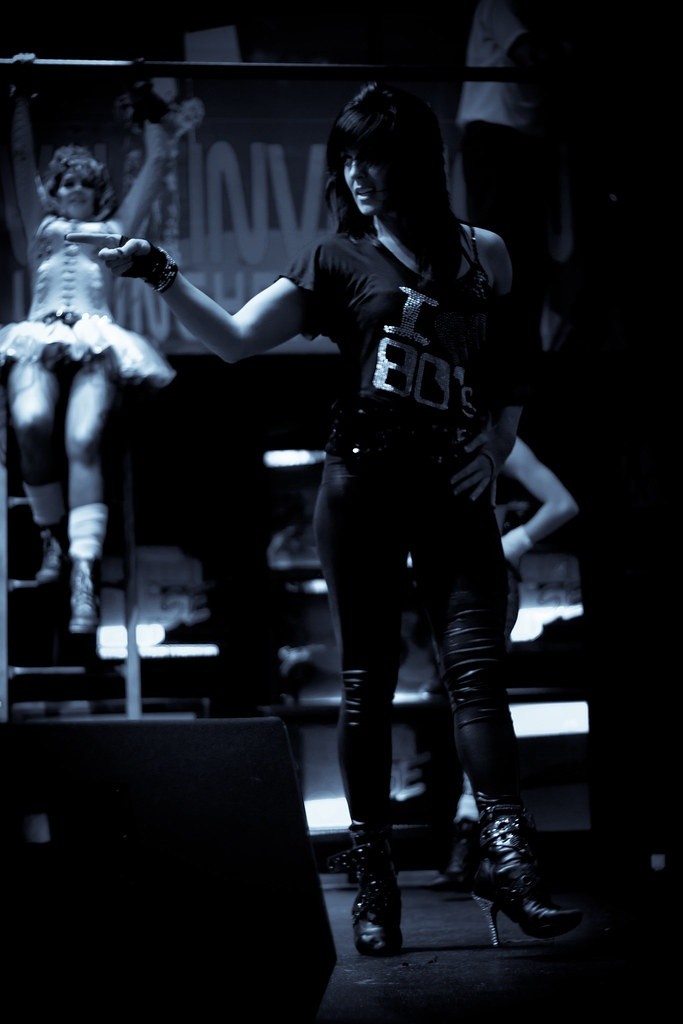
[
  {"xmin": 68, "ymin": 564, "xmax": 102, "ymax": 632},
  {"xmin": 34, "ymin": 519, "xmax": 65, "ymax": 584}
]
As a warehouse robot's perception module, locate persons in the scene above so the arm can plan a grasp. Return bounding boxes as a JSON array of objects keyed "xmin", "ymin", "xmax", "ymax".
[
  {"xmin": 455, "ymin": 0, "xmax": 589, "ymax": 298},
  {"xmin": 64, "ymin": 83, "xmax": 581, "ymax": 951},
  {"xmin": 0, "ymin": 51, "xmax": 176, "ymax": 635}
]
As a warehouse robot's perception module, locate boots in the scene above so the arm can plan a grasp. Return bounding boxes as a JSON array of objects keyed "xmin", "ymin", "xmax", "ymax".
[
  {"xmin": 469, "ymin": 805, "xmax": 582, "ymax": 949},
  {"xmin": 324, "ymin": 818, "xmax": 402, "ymax": 956}
]
